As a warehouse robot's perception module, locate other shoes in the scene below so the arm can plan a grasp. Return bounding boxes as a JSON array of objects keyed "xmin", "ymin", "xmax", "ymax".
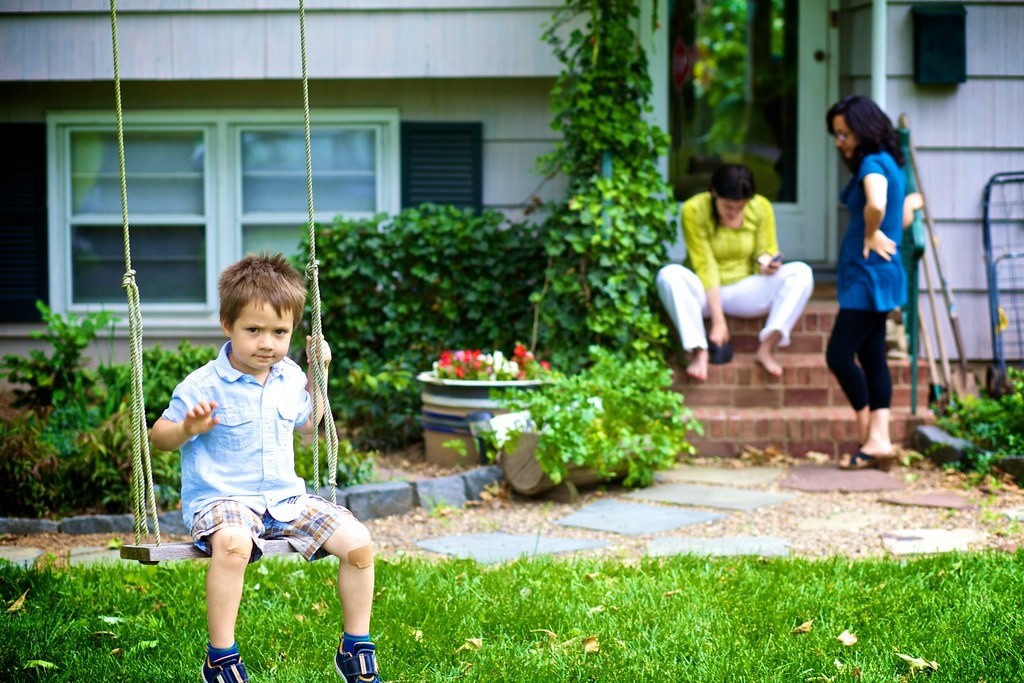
[{"xmin": 708, "ymin": 341, "xmax": 733, "ymax": 364}]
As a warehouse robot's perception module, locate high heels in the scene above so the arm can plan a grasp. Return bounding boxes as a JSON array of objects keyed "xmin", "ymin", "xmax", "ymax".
[{"xmin": 839, "ymin": 445, "xmax": 892, "ymax": 472}]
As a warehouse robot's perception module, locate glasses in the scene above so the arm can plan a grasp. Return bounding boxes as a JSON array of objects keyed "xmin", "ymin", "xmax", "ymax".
[{"xmin": 836, "ymin": 131, "xmax": 853, "ymax": 142}]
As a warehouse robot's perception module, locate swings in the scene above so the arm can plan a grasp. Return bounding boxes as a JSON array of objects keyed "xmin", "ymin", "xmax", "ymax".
[{"xmin": 110, "ymin": 2, "xmax": 340, "ymax": 568}]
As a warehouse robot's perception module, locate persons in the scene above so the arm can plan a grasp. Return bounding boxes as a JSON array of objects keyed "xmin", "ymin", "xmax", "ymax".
[
  {"xmin": 657, "ymin": 163, "xmax": 814, "ymax": 378},
  {"xmin": 826, "ymin": 95, "xmax": 924, "ymax": 472},
  {"xmin": 151, "ymin": 250, "xmax": 379, "ymax": 683}
]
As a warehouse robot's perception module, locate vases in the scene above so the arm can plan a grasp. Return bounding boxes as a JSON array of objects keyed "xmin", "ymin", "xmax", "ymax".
[{"xmin": 417, "ymin": 370, "xmax": 555, "ymax": 469}]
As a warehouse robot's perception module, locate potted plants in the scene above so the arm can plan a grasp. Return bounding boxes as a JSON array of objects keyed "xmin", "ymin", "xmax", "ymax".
[{"xmin": 442, "ymin": 344, "xmax": 704, "ymax": 496}]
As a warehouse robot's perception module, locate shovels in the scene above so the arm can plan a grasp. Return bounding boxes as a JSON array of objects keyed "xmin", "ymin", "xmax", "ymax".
[{"xmin": 900, "ymin": 111, "xmax": 981, "ymax": 407}]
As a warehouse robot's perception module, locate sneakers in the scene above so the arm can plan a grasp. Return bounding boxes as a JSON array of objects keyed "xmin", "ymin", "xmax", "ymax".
[
  {"xmin": 334, "ymin": 634, "xmax": 380, "ymax": 683},
  {"xmin": 201, "ymin": 647, "xmax": 248, "ymax": 683}
]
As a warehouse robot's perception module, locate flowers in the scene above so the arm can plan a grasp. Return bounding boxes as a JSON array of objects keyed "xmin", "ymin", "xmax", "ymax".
[{"xmin": 433, "ymin": 341, "xmax": 551, "ymax": 380}]
